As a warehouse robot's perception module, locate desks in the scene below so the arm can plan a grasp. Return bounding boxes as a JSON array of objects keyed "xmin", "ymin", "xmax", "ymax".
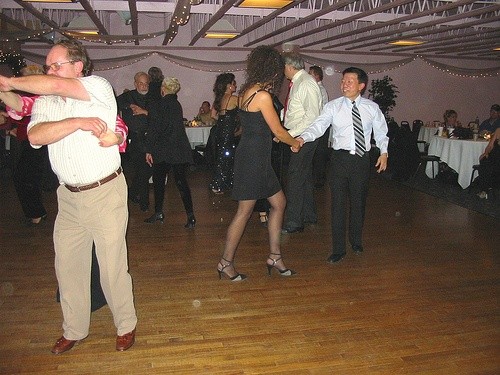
[
  {"xmin": 185, "ymin": 126, "xmax": 212, "ymax": 155},
  {"xmin": 417, "ymin": 126, "xmax": 438, "ymax": 152},
  {"xmin": 425, "ymin": 136, "xmax": 490, "ymax": 189}
]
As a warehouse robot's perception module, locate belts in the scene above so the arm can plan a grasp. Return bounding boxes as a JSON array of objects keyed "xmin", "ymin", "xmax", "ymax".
[{"xmin": 65, "ymin": 167, "xmax": 121, "ymax": 192}]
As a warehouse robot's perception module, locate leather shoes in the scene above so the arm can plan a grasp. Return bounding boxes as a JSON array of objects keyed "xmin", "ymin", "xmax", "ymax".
[
  {"xmin": 51, "ymin": 336, "xmax": 76, "ymax": 353},
  {"xmin": 117, "ymin": 328, "xmax": 135, "ymax": 350}
]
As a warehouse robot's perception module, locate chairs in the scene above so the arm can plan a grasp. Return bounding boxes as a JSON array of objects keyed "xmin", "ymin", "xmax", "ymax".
[
  {"xmin": 195, "ymin": 127, "xmax": 212, "ymax": 158},
  {"xmin": 389, "ymin": 119, "xmax": 500, "ymax": 188}
]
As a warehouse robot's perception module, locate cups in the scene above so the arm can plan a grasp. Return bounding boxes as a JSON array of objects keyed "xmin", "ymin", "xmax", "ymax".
[
  {"xmin": 447, "ymin": 128, "xmax": 455, "ymax": 139},
  {"xmin": 205, "ymin": 121, "xmax": 212, "ymax": 126},
  {"xmin": 472, "ymin": 130, "xmax": 479, "ymax": 141},
  {"xmin": 438, "ymin": 127, "xmax": 443, "ymax": 136}
]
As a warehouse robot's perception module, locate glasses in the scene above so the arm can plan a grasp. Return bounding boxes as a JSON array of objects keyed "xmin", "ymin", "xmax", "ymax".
[{"xmin": 42, "ymin": 61, "xmax": 75, "ymax": 74}]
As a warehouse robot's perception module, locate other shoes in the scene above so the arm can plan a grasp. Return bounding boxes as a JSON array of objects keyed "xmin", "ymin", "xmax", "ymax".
[
  {"xmin": 328, "ymin": 253, "xmax": 348, "ymax": 262},
  {"xmin": 300, "ymin": 221, "xmax": 318, "ymax": 224},
  {"xmin": 352, "ymin": 244, "xmax": 363, "ymax": 252},
  {"xmin": 282, "ymin": 227, "xmax": 304, "ymax": 233}
]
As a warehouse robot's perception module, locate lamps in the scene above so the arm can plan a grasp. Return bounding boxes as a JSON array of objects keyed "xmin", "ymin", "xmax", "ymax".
[
  {"xmin": 67, "ymin": 15, "xmax": 100, "ymax": 34},
  {"xmin": 385, "ymin": 39, "xmax": 427, "ymax": 45},
  {"xmin": 239, "ymin": 0, "xmax": 294, "ymax": 9},
  {"xmin": 202, "ymin": 18, "xmax": 240, "ymax": 39}
]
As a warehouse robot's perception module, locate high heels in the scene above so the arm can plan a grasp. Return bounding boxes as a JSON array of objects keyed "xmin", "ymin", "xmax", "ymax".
[
  {"xmin": 265, "ymin": 256, "xmax": 297, "ymax": 277},
  {"xmin": 144, "ymin": 213, "xmax": 165, "ymax": 224},
  {"xmin": 259, "ymin": 211, "xmax": 268, "ymax": 223},
  {"xmin": 185, "ymin": 215, "xmax": 196, "ymax": 230},
  {"xmin": 29, "ymin": 212, "xmax": 47, "ymax": 225},
  {"xmin": 216, "ymin": 259, "xmax": 247, "ymax": 281}
]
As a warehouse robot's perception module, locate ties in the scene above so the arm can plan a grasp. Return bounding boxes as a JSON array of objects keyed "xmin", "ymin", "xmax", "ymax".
[
  {"xmin": 284, "ymin": 82, "xmax": 294, "ymax": 128},
  {"xmin": 352, "ymin": 101, "xmax": 366, "ymax": 158}
]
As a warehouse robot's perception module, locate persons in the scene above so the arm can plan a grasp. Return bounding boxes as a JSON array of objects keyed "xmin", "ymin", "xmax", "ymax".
[
  {"xmin": 436, "ymin": 104, "xmax": 500, "ymax": 199},
  {"xmin": 0, "ymin": 39, "xmax": 137, "ymax": 354},
  {"xmin": 115, "ymin": 67, "xmax": 196, "ymax": 229},
  {"xmin": 216, "ymin": 45, "xmax": 301, "ymax": 281},
  {"xmin": 291, "ymin": 68, "xmax": 388, "ymax": 263},
  {"xmin": 198, "ymin": 53, "xmax": 329, "ymax": 234}
]
